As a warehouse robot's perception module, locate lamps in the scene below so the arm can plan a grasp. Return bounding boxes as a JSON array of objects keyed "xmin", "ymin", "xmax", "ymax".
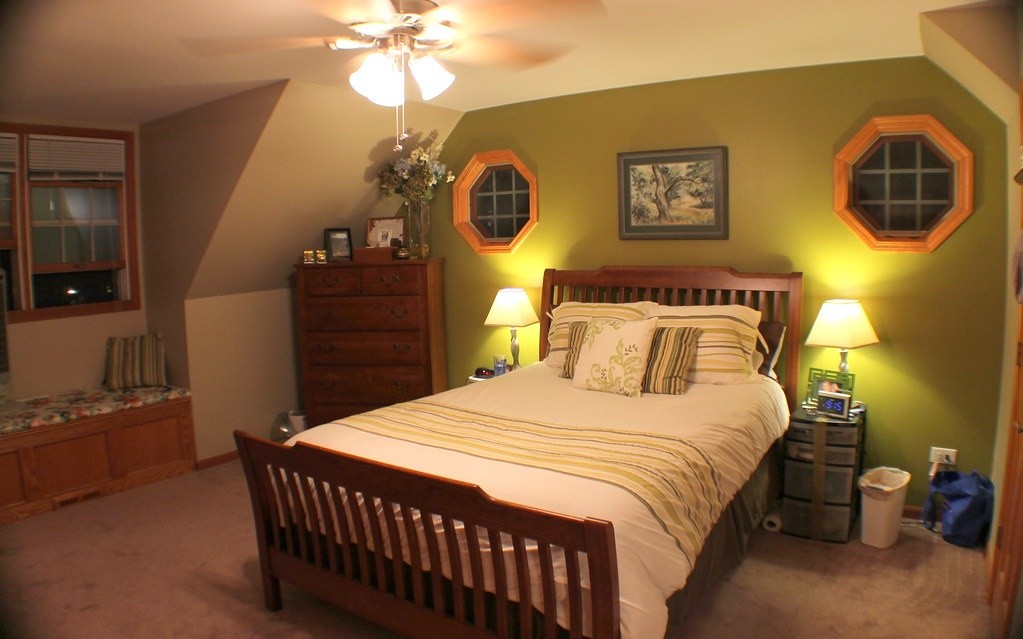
[
  {"xmin": 804, "ymin": 299, "xmax": 880, "ymax": 372},
  {"xmin": 349, "ymin": 49, "xmax": 455, "ymax": 152},
  {"xmin": 95, "ymin": 272, "xmax": 113, "ymax": 295},
  {"xmin": 63, "ymin": 273, "xmax": 80, "ymax": 296},
  {"xmin": 484, "ymin": 289, "xmax": 540, "ymax": 371}
]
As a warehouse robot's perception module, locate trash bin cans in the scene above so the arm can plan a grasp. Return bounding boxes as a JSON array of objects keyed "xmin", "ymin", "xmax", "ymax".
[
  {"xmin": 289, "ymin": 410, "xmax": 307, "ymax": 433},
  {"xmin": 857, "ymin": 466, "xmax": 912, "ymax": 549}
]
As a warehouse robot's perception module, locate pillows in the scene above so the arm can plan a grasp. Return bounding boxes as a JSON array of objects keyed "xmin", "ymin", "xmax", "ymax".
[
  {"xmin": 543, "ymin": 301, "xmax": 788, "ymax": 398},
  {"xmin": 106, "ymin": 331, "xmax": 168, "ymax": 389}
]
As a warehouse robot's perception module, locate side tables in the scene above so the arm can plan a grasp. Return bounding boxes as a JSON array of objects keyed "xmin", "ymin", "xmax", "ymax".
[{"xmin": 781, "ymin": 405, "xmax": 864, "ymax": 544}]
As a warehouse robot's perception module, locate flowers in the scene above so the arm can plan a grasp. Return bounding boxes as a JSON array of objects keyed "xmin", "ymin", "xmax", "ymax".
[{"xmin": 376, "ymin": 146, "xmax": 455, "ymax": 242}]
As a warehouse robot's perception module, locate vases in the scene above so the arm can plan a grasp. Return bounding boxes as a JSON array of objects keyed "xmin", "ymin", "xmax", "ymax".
[{"xmin": 408, "ymin": 201, "xmax": 431, "ymax": 257}]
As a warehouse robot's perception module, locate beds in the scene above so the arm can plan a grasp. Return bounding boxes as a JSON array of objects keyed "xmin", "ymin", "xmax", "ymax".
[{"xmin": 232, "ymin": 257, "xmax": 804, "ymax": 639}]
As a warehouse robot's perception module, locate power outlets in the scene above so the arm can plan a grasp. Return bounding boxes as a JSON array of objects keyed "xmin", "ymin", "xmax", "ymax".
[{"xmin": 930, "ymin": 447, "xmax": 958, "ymax": 465}]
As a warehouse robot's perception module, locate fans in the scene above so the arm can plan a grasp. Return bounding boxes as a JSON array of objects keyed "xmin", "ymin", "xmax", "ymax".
[{"xmin": 335, "ymin": 0, "xmax": 455, "ymax": 56}]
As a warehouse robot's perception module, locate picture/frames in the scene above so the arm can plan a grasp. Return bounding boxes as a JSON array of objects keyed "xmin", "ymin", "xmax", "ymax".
[
  {"xmin": 617, "ymin": 146, "xmax": 729, "ymax": 240},
  {"xmin": 324, "ymin": 228, "xmax": 353, "ymax": 262},
  {"xmin": 806, "ymin": 367, "xmax": 856, "ymax": 409}
]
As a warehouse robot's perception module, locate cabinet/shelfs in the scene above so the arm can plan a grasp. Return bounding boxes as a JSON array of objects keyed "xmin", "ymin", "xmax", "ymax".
[
  {"xmin": 292, "ymin": 254, "xmax": 446, "ymax": 428},
  {"xmin": 0, "ymin": 383, "xmax": 197, "ymax": 520}
]
